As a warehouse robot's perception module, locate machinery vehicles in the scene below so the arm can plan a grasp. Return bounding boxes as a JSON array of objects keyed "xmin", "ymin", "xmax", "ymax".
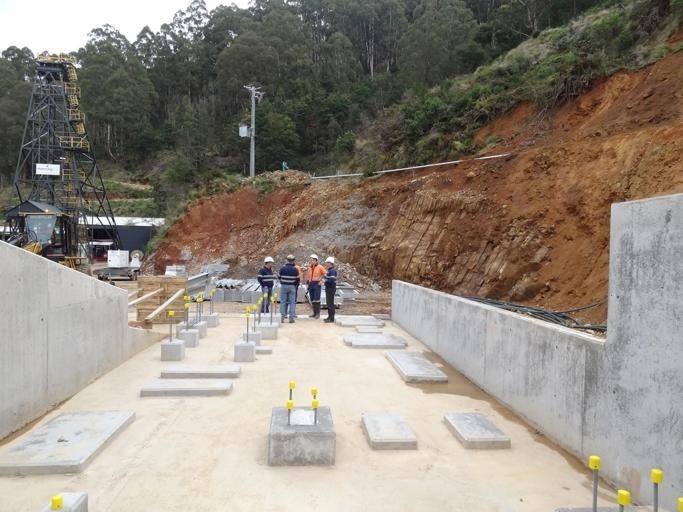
[{"xmin": 10, "ymin": 211, "xmax": 92, "ymax": 276}]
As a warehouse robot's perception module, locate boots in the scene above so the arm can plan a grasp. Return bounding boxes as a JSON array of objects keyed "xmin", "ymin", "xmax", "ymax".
[{"xmin": 309, "ymin": 302, "xmax": 335, "ymax": 323}]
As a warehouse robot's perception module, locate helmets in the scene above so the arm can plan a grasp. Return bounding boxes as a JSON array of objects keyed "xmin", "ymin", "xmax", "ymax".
[
  {"xmin": 287, "ymin": 254, "xmax": 296, "ymax": 260},
  {"xmin": 263, "ymin": 256, "xmax": 274, "ymax": 263},
  {"xmin": 310, "ymin": 253, "xmax": 319, "ymax": 261},
  {"xmin": 325, "ymin": 256, "xmax": 335, "ymax": 264}
]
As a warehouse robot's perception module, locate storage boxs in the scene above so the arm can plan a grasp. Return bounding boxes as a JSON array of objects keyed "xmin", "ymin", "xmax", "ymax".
[{"xmin": 136, "ymin": 275, "xmax": 188, "ymax": 325}]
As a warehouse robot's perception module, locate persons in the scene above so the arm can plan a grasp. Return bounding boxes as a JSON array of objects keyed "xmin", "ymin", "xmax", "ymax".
[
  {"xmin": 306, "ymin": 254, "xmax": 326, "ymax": 319},
  {"xmin": 257, "ymin": 257, "xmax": 275, "ymax": 313},
  {"xmin": 277, "ymin": 255, "xmax": 300, "ymax": 323},
  {"xmin": 323, "ymin": 257, "xmax": 337, "ymax": 323},
  {"xmin": 285, "ymin": 260, "xmax": 301, "ymax": 318}
]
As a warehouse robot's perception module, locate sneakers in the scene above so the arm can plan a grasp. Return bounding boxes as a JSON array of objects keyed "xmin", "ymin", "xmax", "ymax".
[{"xmin": 281, "ymin": 320, "xmax": 296, "ymax": 323}]
